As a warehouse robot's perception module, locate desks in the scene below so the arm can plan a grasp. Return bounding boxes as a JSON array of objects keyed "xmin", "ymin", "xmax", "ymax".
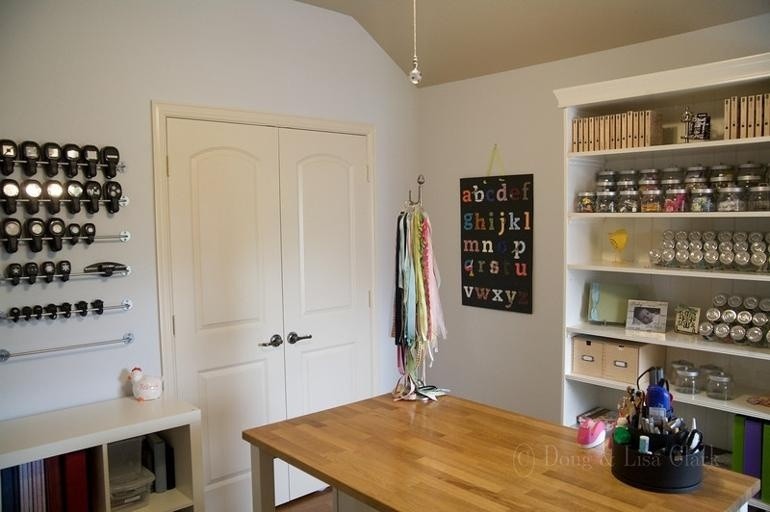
[{"xmin": 242, "ymin": 392, "xmax": 760, "ymax": 512}]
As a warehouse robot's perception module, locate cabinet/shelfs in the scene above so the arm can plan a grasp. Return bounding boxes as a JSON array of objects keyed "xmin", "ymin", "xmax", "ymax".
[
  {"xmin": 0, "ymin": 393, "xmax": 205, "ymax": 512},
  {"xmin": 552, "ymin": 50, "xmax": 770, "ymax": 511}
]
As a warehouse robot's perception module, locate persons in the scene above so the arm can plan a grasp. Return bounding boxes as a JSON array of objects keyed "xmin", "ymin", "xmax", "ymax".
[{"xmin": 634, "ymin": 306, "xmax": 657, "ymax": 325}]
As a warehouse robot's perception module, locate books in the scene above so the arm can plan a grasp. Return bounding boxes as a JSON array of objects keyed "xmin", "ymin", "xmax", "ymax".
[
  {"xmin": 576, "ymin": 405, "xmax": 617, "ymax": 429},
  {"xmin": 151, "ymin": 434, "xmax": 166, "ymax": 493},
  {"xmin": 1, "ymin": 452, "xmax": 92, "ymax": 511},
  {"xmin": 166, "ymin": 444, "xmax": 177, "ymax": 490},
  {"xmin": 571, "ymin": 109, "xmax": 663, "ymax": 150},
  {"xmin": 722, "ymin": 93, "xmax": 770, "ymax": 138}
]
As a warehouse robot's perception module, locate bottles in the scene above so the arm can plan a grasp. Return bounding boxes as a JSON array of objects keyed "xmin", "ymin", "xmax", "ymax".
[
  {"xmin": 670, "ymin": 359, "xmax": 736, "ymax": 401},
  {"xmin": 574, "ymin": 160, "xmax": 770, "ymax": 213}
]
